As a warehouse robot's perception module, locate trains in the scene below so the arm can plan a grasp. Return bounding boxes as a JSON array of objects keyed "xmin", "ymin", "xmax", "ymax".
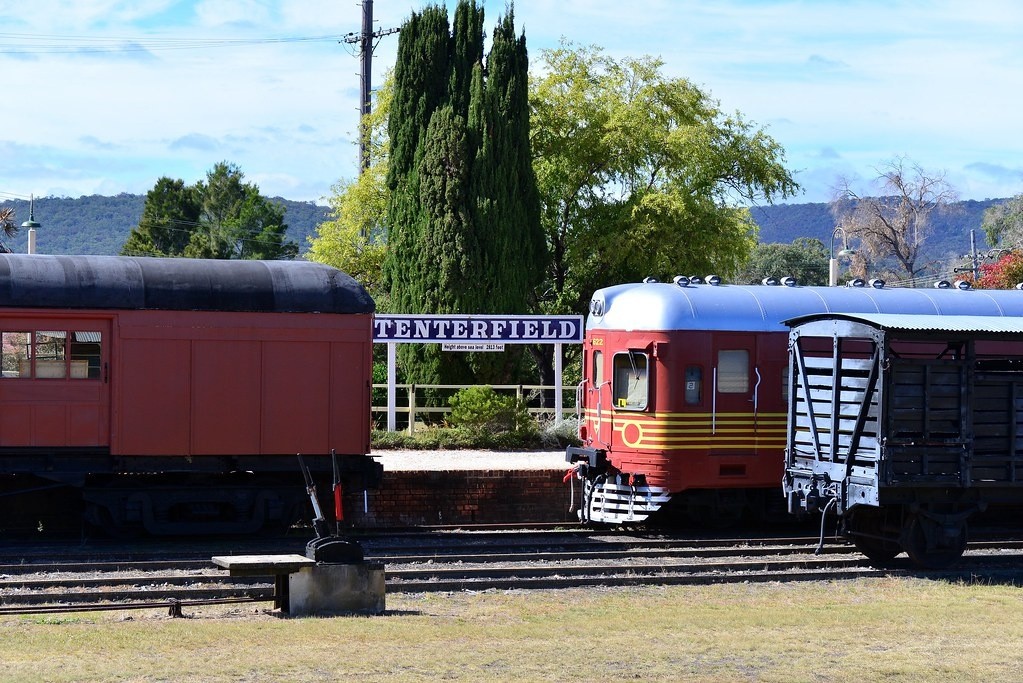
[
  {"xmin": 779, "ymin": 313, "xmax": 1023, "ymax": 568},
  {"xmin": 0, "ymin": 252, "xmax": 384, "ymax": 541},
  {"xmin": 564, "ymin": 274, "xmax": 1023, "ymax": 523}
]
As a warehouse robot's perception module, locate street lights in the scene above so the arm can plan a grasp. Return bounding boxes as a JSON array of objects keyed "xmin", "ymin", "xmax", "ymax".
[
  {"xmin": 829, "ymin": 228, "xmax": 856, "ymax": 286},
  {"xmin": 21, "ymin": 192, "xmax": 42, "ymax": 254}
]
